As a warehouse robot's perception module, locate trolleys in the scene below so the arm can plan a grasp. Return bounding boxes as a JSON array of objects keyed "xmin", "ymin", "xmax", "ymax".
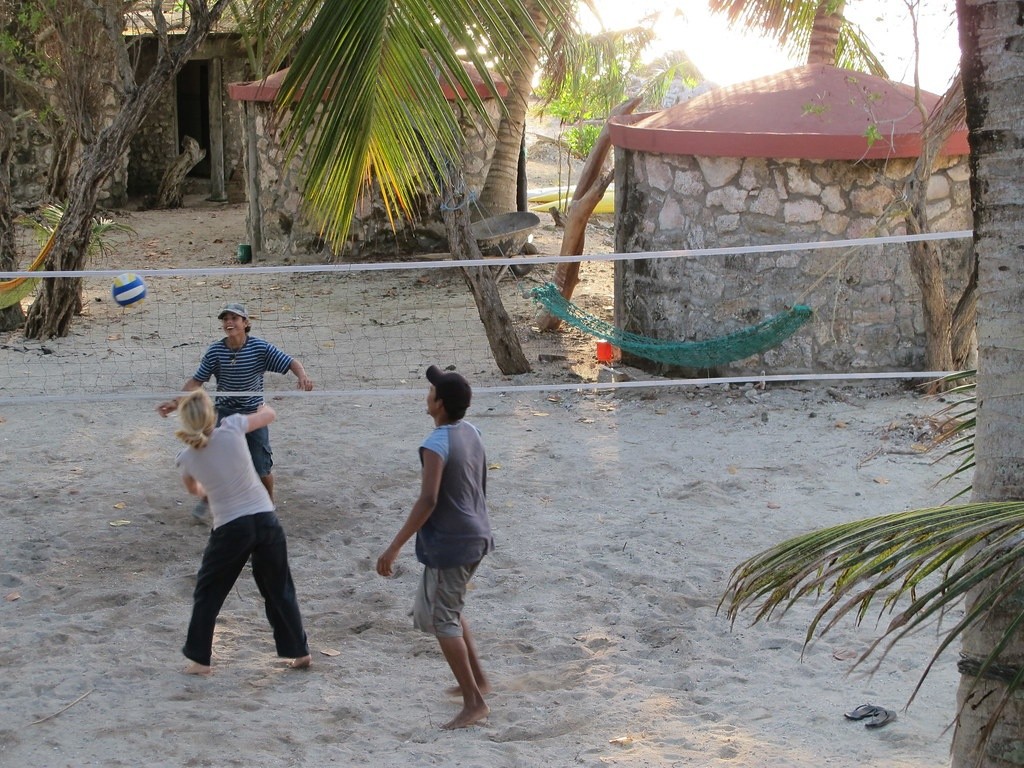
[{"xmin": 470, "ymin": 212, "xmax": 541, "ymax": 286}]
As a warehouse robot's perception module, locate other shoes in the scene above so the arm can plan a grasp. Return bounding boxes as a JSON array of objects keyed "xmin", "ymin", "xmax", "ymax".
[{"xmin": 192, "ymin": 501, "xmax": 207, "ymax": 520}]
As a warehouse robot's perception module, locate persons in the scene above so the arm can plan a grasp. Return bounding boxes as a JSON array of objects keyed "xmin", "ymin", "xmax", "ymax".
[
  {"xmin": 377, "ymin": 365, "xmax": 496, "ymax": 731},
  {"xmin": 156, "ymin": 302, "xmax": 315, "ymax": 521},
  {"xmin": 175, "ymin": 390, "xmax": 312, "ymax": 675}
]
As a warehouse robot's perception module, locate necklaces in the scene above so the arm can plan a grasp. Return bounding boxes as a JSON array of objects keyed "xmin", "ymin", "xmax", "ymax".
[{"xmin": 229, "ymin": 336, "xmax": 246, "ymax": 365}]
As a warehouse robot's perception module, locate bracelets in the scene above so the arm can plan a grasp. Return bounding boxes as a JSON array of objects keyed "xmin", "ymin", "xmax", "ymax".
[{"xmin": 173, "ymin": 399, "xmax": 180, "ymax": 406}]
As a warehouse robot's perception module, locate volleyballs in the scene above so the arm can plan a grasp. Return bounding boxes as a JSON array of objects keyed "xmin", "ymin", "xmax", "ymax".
[{"xmin": 112, "ymin": 273, "xmax": 146, "ymax": 308}]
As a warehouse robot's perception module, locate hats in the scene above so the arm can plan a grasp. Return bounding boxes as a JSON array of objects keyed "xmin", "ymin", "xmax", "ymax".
[
  {"xmin": 217, "ymin": 303, "xmax": 248, "ymax": 319},
  {"xmin": 426, "ymin": 364, "xmax": 472, "ymax": 410}
]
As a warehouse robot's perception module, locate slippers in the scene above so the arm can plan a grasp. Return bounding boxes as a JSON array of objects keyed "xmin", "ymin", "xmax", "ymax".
[
  {"xmin": 865, "ymin": 709, "xmax": 896, "ymax": 727},
  {"xmin": 844, "ymin": 704, "xmax": 884, "ymax": 720}
]
